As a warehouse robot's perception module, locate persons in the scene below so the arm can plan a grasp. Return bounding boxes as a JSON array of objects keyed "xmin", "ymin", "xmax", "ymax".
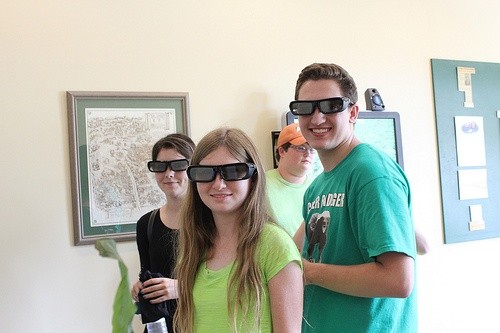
[
  {"xmin": 258, "ymin": 123, "xmax": 317, "ymax": 239},
  {"xmin": 170, "ymin": 126, "xmax": 305, "ymax": 333},
  {"xmin": 129, "ymin": 133, "xmax": 201, "ymax": 333},
  {"xmin": 287, "ymin": 62, "xmax": 421, "ymax": 333}
]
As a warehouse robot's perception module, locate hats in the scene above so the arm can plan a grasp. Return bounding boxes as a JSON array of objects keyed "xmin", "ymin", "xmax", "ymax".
[{"xmin": 277, "ymin": 122, "xmax": 307, "ymax": 147}]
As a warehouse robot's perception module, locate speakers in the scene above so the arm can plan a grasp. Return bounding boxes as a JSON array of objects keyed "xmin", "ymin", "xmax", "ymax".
[{"xmin": 365, "ymin": 88, "xmax": 386, "ymax": 112}]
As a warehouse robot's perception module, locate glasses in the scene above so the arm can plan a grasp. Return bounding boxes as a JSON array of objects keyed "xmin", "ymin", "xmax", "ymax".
[
  {"xmin": 289, "ymin": 96, "xmax": 353, "ymax": 117},
  {"xmin": 147, "ymin": 158, "xmax": 190, "ymax": 173},
  {"xmin": 186, "ymin": 162, "xmax": 256, "ymax": 182},
  {"xmin": 290, "ymin": 145, "xmax": 315, "ymax": 154}
]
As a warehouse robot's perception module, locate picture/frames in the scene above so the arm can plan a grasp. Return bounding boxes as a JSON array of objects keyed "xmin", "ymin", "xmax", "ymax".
[{"xmin": 66, "ymin": 90, "xmax": 192, "ymax": 249}]
